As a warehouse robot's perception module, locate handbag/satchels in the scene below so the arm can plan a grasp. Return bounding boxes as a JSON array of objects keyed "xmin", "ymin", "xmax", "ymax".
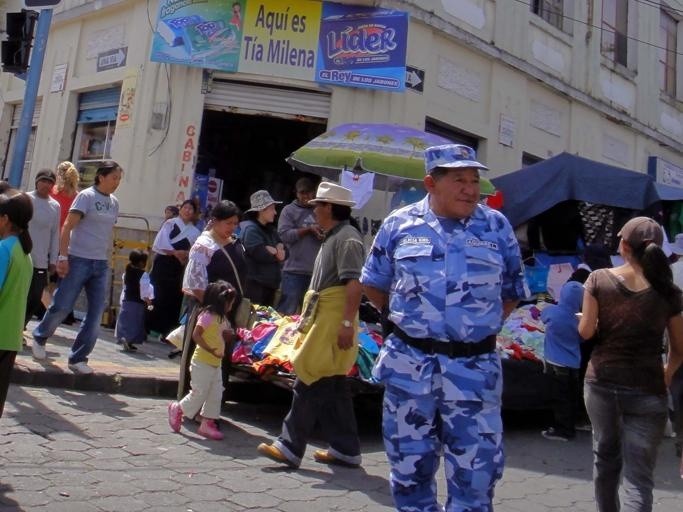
[{"xmin": 235, "ymin": 297, "xmax": 256, "ymax": 331}]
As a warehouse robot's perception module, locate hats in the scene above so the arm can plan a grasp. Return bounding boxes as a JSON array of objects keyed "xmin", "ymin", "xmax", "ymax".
[
  {"xmin": 295, "ymin": 177, "xmax": 313, "ymax": 192},
  {"xmin": 307, "ymin": 181, "xmax": 356, "ymax": 207},
  {"xmin": 424, "ymin": 144, "xmax": 490, "ymax": 173},
  {"xmin": 617, "ymin": 217, "xmax": 664, "ymax": 248},
  {"xmin": 35, "ymin": 168, "xmax": 56, "ymax": 184},
  {"xmin": 245, "ymin": 190, "xmax": 284, "ymax": 213}
]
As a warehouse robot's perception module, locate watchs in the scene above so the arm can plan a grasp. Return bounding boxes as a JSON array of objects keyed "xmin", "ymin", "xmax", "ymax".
[
  {"xmin": 58, "ymin": 256, "xmax": 68, "ymax": 261},
  {"xmin": 340, "ymin": 320, "xmax": 355, "ymax": 328}
]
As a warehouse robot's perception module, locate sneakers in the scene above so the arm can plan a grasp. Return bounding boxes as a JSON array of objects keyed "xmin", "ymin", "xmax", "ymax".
[
  {"xmin": 68, "ymin": 361, "xmax": 94, "ymax": 375},
  {"xmin": 168, "ymin": 402, "xmax": 183, "ymax": 433},
  {"xmin": 257, "ymin": 442, "xmax": 298, "ymax": 469},
  {"xmin": 121, "ymin": 336, "xmax": 137, "ymax": 351},
  {"xmin": 197, "ymin": 420, "xmax": 224, "ymax": 440},
  {"xmin": 313, "ymin": 450, "xmax": 359, "ymax": 467},
  {"xmin": 32, "ymin": 339, "xmax": 46, "ymax": 359},
  {"xmin": 541, "ymin": 430, "xmax": 570, "ymax": 442}
]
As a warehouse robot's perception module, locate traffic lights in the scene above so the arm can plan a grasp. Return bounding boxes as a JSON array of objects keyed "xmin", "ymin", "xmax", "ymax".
[{"xmin": 0, "ymin": 7, "xmax": 37, "ymax": 74}]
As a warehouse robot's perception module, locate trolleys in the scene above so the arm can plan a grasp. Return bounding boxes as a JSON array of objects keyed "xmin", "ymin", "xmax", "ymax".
[{"xmin": 102, "ymin": 214, "xmax": 154, "ymax": 330}]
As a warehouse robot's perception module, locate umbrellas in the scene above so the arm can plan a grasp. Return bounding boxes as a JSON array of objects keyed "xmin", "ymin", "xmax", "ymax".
[{"xmin": 284, "ymin": 121, "xmax": 496, "ymax": 240}]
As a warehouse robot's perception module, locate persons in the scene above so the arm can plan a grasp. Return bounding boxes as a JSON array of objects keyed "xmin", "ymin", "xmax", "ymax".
[
  {"xmin": 359, "ymin": 143, "xmax": 531, "ymax": 512},
  {"xmin": 0, "ymin": 187, "xmax": 33, "ymax": 419},
  {"xmin": 229, "ymin": 2, "xmax": 241, "ymax": 32},
  {"xmin": 258, "ymin": 182, "xmax": 366, "ymax": 469},
  {"xmin": 32, "ymin": 161, "xmax": 124, "ymax": 374},
  {"xmin": 48, "ymin": 160, "xmax": 80, "ymax": 326},
  {"xmin": 22, "ymin": 168, "xmax": 62, "ymax": 347}
]
